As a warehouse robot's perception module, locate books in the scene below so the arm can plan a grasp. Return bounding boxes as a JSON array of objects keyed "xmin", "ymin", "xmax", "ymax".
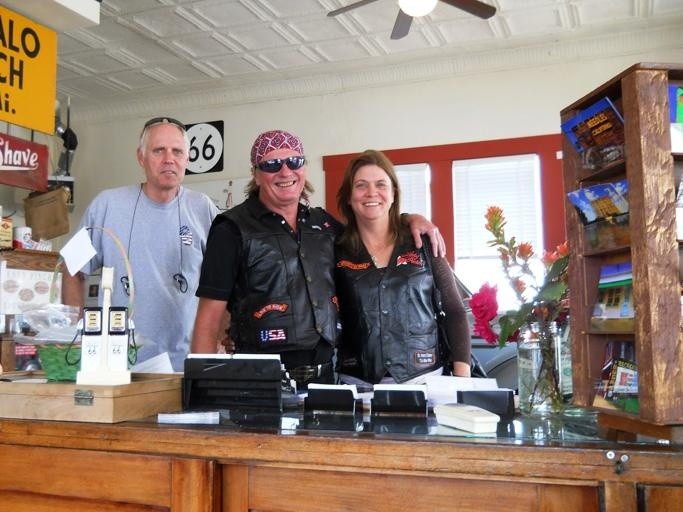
[
  {"xmin": 561, "ymin": 98, "xmax": 624, "ymax": 169},
  {"xmin": 669, "ymin": 87, "xmax": 682, "ymax": 238},
  {"xmin": 592, "ymin": 261, "xmax": 634, "ymax": 318},
  {"xmin": 567, "ymin": 180, "xmax": 630, "ymax": 250},
  {"xmin": 595, "ymin": 341, "xmax": 638, "ymax": 412}
]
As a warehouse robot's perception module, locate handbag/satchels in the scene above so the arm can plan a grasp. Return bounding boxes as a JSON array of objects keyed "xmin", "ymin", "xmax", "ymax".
[
  {"xmin": 23, "ymin": 184, "xmax": 70, "ymax": 243},
  {"xmin": 436, "ymin": 292, "xmax": 487, "ymax": 378}
]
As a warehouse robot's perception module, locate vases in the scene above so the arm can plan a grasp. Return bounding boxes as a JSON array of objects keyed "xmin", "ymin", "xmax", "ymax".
[{"xmin": 516, "ymin": 321, "xmax": 567, "ymax": 421}]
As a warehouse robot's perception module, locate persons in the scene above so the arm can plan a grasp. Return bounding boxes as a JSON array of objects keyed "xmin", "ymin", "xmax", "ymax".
[
  {"xmin": 219, "ymin": 148, "xmax": 471, "ymax": 394},
  {"xmin": 189, "ymin": 129, "xmax": 447, "ymax": 394},
  {"xmin": 68, "ymin": 114, "xmax": 225, "ymax": 377}
]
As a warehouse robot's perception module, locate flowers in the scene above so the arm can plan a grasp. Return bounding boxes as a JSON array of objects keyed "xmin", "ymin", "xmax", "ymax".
[{"xmin": 483, "ymin": 204, "xmax": 572, "ymax": 413}]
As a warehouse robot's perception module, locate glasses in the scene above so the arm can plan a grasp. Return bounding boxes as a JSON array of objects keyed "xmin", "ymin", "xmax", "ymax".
[
  {"xmin": 255, "ymin": 157, "xmax": 305, "ymax": 172},
  {"xmin": 140, "ymin": 117, "xmax": 186, "ymax": 139}
]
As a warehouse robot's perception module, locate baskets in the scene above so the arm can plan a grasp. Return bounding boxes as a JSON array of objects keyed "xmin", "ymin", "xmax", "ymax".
[{"xmin": 36, "ymin": 227, "xmax": 140, "ymax": 381}]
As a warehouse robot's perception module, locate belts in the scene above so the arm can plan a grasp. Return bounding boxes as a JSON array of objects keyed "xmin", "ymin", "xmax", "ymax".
[{"xmin": 289, "ymin": 362, "xmax": 331, "ymax": 383}]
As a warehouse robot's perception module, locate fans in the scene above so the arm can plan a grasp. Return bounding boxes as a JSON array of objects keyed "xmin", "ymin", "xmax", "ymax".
[{"xmin": 327, "ymin": 0, "xmax": 498, "ymax": 39}]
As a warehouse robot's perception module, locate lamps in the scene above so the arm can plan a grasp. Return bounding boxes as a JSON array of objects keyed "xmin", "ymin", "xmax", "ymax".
[{"xmin": 398, "ymin": 0, "xmax": 439, "ymax": 18}]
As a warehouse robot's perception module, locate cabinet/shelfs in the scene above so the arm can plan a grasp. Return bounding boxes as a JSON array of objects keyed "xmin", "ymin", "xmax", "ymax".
[{"xmin": 559, "ymin": 61, "xmax": 683, "ymax": 427}]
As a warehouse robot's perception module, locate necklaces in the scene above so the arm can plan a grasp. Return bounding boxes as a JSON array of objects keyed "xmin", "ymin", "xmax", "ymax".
[{"xmin": 362, "ymin": 234, "xmax": 390, "ymax": 266}]
